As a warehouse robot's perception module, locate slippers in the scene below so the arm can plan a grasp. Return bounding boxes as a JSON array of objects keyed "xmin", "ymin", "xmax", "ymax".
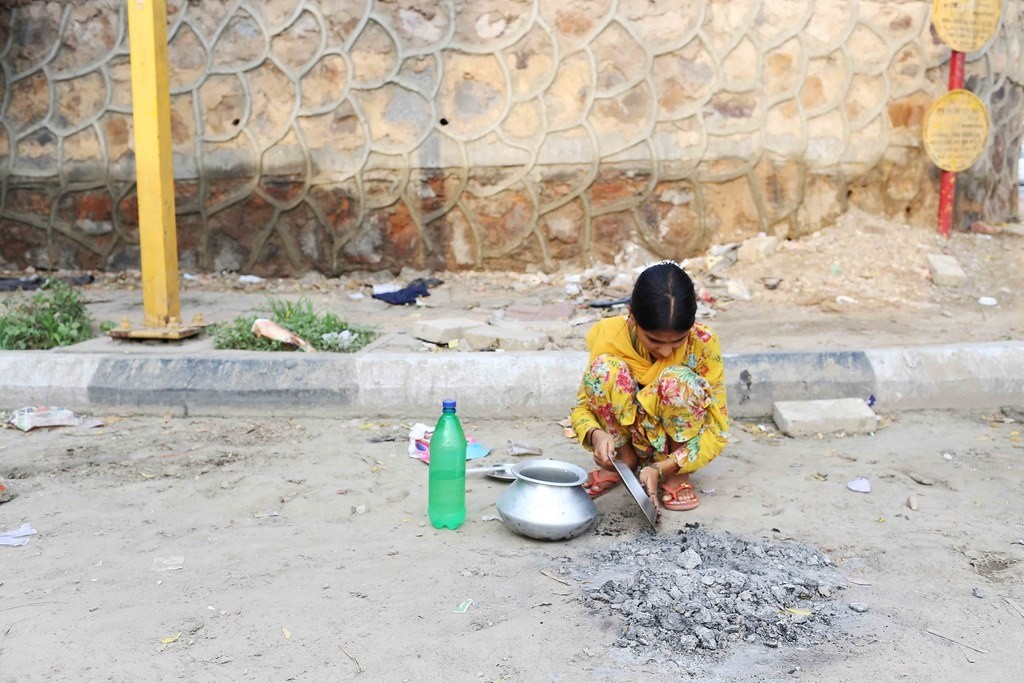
[
  {"xmin": 582, "ymin": 470, "xmax": 620, "ymax": 500},
  {"xmin": 660, "ymin": 482, "xmax": 699, "ymax": 511}
]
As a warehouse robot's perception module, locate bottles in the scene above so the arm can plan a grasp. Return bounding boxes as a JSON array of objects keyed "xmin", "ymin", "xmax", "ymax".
[
  {"xmin": 495, "ymin": 460, "xmax": 600, "ymax": 542},
  {"xmin": 428, "ymin": 399, "xmax": 466, "ymax": 529}
]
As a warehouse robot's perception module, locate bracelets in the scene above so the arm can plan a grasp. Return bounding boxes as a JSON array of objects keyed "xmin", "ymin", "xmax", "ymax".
[
  {"xmin": 641, "ymin": 462, "xmax": 666, "ymax": 482},
  {"xmin": 590, "ymin": 428, "xmax": 603, "ymax": 446}
]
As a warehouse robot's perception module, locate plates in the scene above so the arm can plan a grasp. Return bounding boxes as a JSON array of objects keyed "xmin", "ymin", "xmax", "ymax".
[
  {"xmin": 609, "ymin": 459, "xmax": 655, "ymax": 522},
  {"xmin": 487, "ymin": 463, "xmax": 516, "ymax": 481}
]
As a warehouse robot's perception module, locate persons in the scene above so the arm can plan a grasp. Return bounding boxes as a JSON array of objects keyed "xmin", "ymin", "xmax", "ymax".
[{"xmin": 567, "ymin": 261, "xmax": 730, "ymax": 511}]
